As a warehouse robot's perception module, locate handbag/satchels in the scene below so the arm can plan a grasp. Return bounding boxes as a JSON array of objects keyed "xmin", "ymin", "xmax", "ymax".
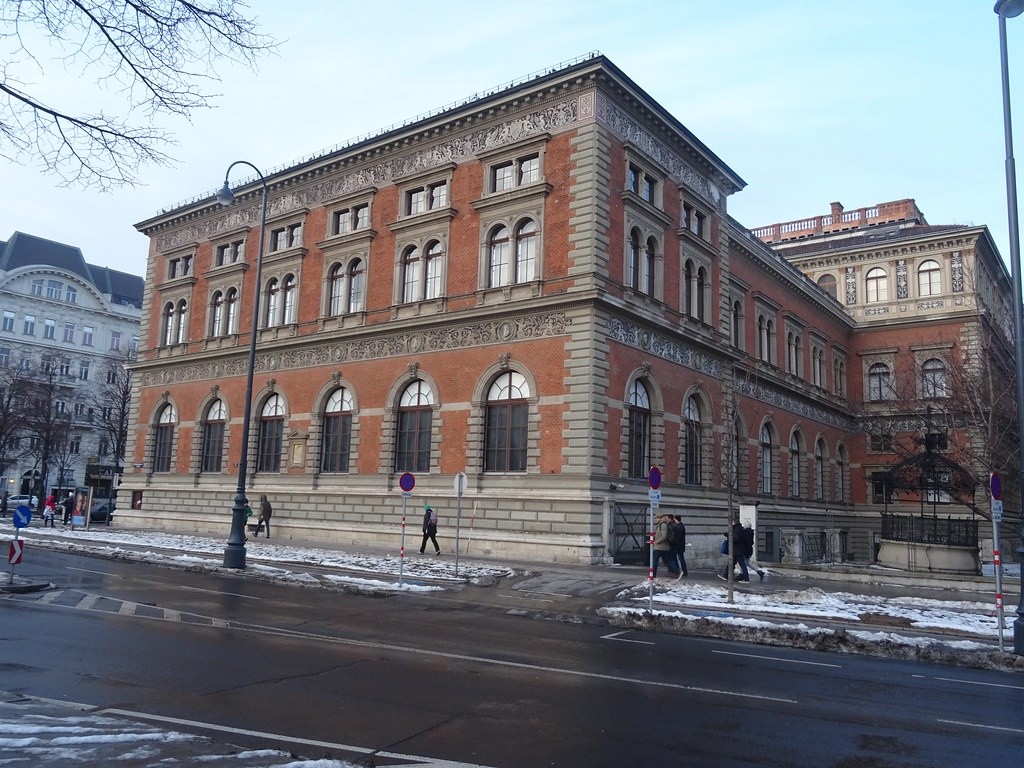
[{"xmin": 721, "ymin": 537, "xmax": 728, "ymax": 554}]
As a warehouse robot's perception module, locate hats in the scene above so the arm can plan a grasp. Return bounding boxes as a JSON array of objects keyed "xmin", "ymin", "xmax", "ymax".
[
  {"xmin": 424, "ymin": 505, "xmax": 430, "ymax": 509},
  {"xmin": 653, "ymin": 512, "xmax": 663, "ymax": 518}
]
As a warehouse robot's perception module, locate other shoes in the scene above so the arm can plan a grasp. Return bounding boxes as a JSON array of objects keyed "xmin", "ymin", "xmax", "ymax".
[
  {"xmin": 417, "ymin": 551, "xmax": 424, "ymax": 555},
  {"xmin": 45, "ymin": 525, "xmax": 56, "ymax": 528},
  {"xmin": 251, "ymin": 533, "xmax": 257, "ymax": 537},
  {"xmin": 265, "ymin": 534, "xmax": 270, "ymax": 538},
  {"xmin": 435, "ymin": 551, "xmax": 440, "ymax": 556},
  {"xmin": 245, "ymin": 537, "xmax": 248, "ymax": 542},
  {"xmin": 3, "ymin": 515, "xmax": 6, "ymax": 518},
  {"xmin": 681, "ymin": 571, "xmax": 687, "ymax": 576}
]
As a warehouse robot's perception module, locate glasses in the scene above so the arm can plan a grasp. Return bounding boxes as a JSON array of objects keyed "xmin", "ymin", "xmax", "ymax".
[{"xmin": 655, "ymin": 517, "xmax": 657, "ymax": 519}]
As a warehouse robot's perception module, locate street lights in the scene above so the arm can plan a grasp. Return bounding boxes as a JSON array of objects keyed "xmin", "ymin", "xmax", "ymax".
[
  {"xmin": 994, "ymin": 0, "xmax": 1024, "ymax": 655},
  {"xmin": 218, "ymin": 160, "xmax": 268, "ymax": 571}
]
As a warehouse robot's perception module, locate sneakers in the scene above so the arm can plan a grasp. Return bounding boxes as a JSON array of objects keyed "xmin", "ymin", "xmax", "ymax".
[
  {"xmin": 717, "ymin": 572, "xmax": 727, "ymax": 581},
  {"xmin": 673, "ymin": 570, "xmax": 684, "ymax": 582},
  {"xmin": 738, "ymin": 577, "xmax": 750, "ymax": 583}
]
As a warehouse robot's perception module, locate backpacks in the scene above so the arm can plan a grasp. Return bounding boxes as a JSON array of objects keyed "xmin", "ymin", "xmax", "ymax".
[
  {"xmin": 660, "ymin": 521, "xmax": 675, "ymax": 543},
  {"xmin": 427, "ymin": 511, "xmax": 437, "ymax": 526}
]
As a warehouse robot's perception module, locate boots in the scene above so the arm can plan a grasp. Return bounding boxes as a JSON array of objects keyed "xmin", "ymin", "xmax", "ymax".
[
  {"xmin": 757, "ymin": 570, "xmax": 765, "ymax": 582},
  {"xmin": 734, "ymin": 573, "xmax": 743, "ymax": 581}
]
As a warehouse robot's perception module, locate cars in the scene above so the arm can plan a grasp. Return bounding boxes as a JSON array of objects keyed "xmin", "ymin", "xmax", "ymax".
[
  {"xmin": 90, "ymin": 502, "xmax": 116, "ymax": 522},
  {"xmin": 0, "ymin": 496, "xmax": 39, "ymax": 508}
]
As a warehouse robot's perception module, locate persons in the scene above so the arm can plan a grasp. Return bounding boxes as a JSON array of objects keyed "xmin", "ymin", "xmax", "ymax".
[
  {"xmin": 736, "ymin": 520, "xmax": 765, "ymax": 581},
  {"xmin": 0, "ymin": 491, "xmax": 10, "ymax": 518},
  {"xmin": 252, "ymin": 494, "xmax": 272, "ymax": 538},
  {"xmin": 417, "ymin": 504, "xmax": 440, "ymax": 556},
  {"xmin": 646, "ymin": 513, "xmax": 688, "ymax": 581},
  {"xmin": 73, "ymin": 492, "xmax": 87, "ymax": 516},
  {"xmin": 643, "ymin": 333, "xmax": 648, "ymax": 346},
  {"xmin": 336, "ymin": 348, "xmax": 342, "ymax": 359},
  {"xmin": 503, "ymin": 324, "xmax": 510, "ymax": 337},
  {"xmin": 61, "ymin": 492, "xmax": 75, "ymax": 525},
  {"xmin": 412, "ymin": 337, "xmax": 419, "ymax": 350},
  {"xmin": 165, "ymin": 372, "xmax": 171, "ymax": 381},
  {"xmin": 214, "ymin": 365, "xmax": 221, "ymax": 376},
  {"xmin": 269, "ymin": 357, "xmax": 276, "ymax": 368},
  {"xmin": 45, "ymin": 495, "xmax": 56, "ymax": 528},
  {"xmin": 718, "ymin": 515, "xmax": 749, "ymax": 584}
]
